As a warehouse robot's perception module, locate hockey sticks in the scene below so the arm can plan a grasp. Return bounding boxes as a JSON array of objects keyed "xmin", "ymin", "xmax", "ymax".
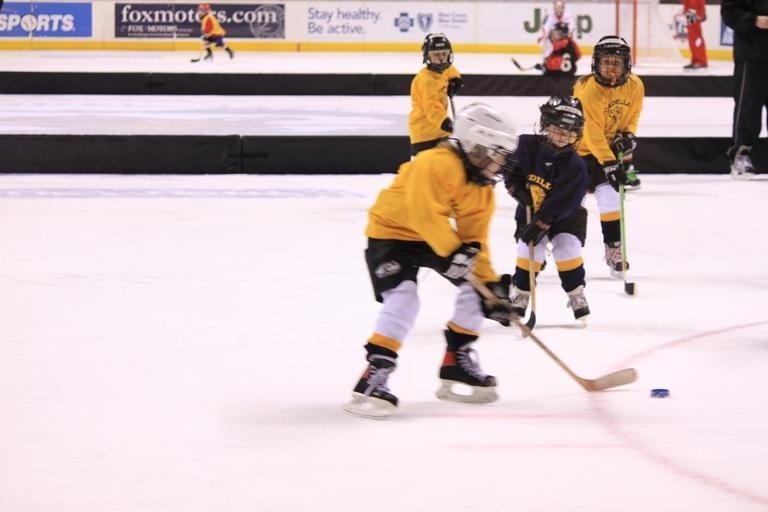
[
  {"xmin": 617, "ymin": 151, "xmax": 634, "ymax": 297},
  {"xmin": 522, "ymin": 207, "xmax": 537, "ymax": 339},
  {"xmin": 463, "ymin": 270, "xmax": 635, "ymax": 391},
  {"xmin": 190, "ymin": 47, "xmax": 202, "ymax": 62},
  {"xmin": 510, "ymin": 57, "xmax": 544, "ymax": 72}
]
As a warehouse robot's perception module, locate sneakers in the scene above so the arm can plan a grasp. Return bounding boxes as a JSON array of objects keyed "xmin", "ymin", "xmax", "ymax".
[
  {"xmin": 604, "ymin": 241, "xmax": 628, "ymax": 271},
  {"xmin": 684, "ymin": 63, "xmax": 707, "ymax": 69},
  {"xmin": 353, "ymin": 352, "xmax": 398, "ymax": 408},
  {"xmin": 726, "ymin": 146, "xmax": 753, "ymax": 174},
  {"xmin": 566, "ymin": 284, "xmax": 590, "ymax": 318},
  {"xmin": 510, "ymin": 286, "xmax": 531, "ymax": 316},
  {"xmin": 440, "ymin": 346, "xmax": 496, "ymax": 388},
  {"xmin": 230, "ymin": 52, "xmax": 233, "ymax": 58},
  {"xmin": 204, "ymin": 55, "xmax": 213, "ymax": 60}
]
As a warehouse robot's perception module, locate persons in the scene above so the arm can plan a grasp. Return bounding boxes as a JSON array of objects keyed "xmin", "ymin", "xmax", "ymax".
[
  {"xmin": 501, "ymin": 95, "xmax": 590, "ymax": 320},
  {"xmin": 536, "ymin": 1, "xmax": 575, "ymax": 57},
  {"xmin": 720, "ymin": 0, "xmax": 768, "ymax": 180},
  {"xmin": 537, "ymin": 22, "xmax": 582, "ymax": 93},
  {"xmin": 198, "ymin": 3, "xmax": 233, "ymax": 59},
  {"xmin": 341, "ymin": 102, "xmax": 519, "ymax": 419},
  {"xmin": 682, "ymin": 1, "xmax": 707, "ymax": 68},
  {"xmin": 408, "ymin": 32, "xmax": 462, "ymax": 155},
  {"xmin": 574, "ymin": 36, "xmax": 645, "ymax": 270}
]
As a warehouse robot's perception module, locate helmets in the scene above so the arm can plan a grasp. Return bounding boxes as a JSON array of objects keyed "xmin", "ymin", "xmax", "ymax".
[
  {"xmin": 539, "ymin": 94, "xmax": 584, "ymax": 154},
  {"xmin": 199, "ymin": 2, "xmax": 210, "ymax": 14},
  {"xmin": 421, "ymin": 33, "xmax": 453, "ymax": 74},
  {"xmin": 553, "ymin": 22, "xmax": 569, "ymax": 34},
  {"xmin": 448, "ymin": 104, "xmax": 519, "ymax": 185},
  {"xmin": 591, "ymin": 35, "xmax": 631, "ymax": 87}
]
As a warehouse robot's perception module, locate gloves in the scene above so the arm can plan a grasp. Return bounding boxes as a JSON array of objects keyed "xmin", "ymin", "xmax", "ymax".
[
  {"xmin": 603, "ymin": 162, "xmax": 640, "ymax": 193},
  {"xmin": 611, "ymin": 132, "xmax": 638, "ymax": 158},
  {"xmin": 447, "ymin": 78, "xmax": 464, "ymax": 99},
  {"xmin": 481, "ymin": 274, "xmax": 512, "ymax": 327},
  {"xmin": 508, "ymin": 184, "xmax": 534, "ymax": 210},
  {"xmin": 520, "ymin": 211, "xmax": 555, "ymax": 246},
  {"xmin": 440, "ymin": 242, "xmax": 481, "ymax": 281}
]
